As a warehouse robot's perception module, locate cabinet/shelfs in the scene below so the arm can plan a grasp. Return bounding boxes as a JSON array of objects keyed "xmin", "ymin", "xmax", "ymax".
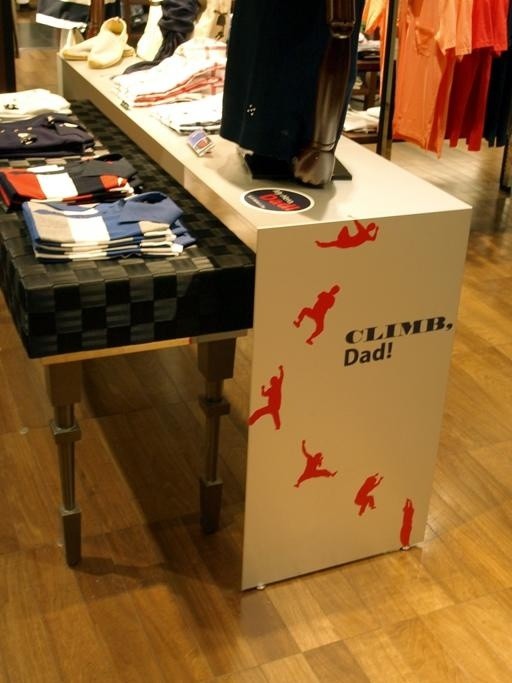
[{"xmin": 346, "ymin": 0, "xmax": 403, "ymax": 162}]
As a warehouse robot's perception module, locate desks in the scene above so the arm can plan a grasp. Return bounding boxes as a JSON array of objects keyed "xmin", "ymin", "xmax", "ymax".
[{"xmin": 42, "ymin": 324, "xmax": 252, "ymax": 570}]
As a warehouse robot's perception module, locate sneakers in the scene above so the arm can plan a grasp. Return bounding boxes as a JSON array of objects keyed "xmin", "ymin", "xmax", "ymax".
[
  {"xmin": 88, "ymin": 16, "xmax": 129, "ymax": 69},
  {"xmin": 63, "ymin": 35, "xmax": 135, "ymax": 61}
]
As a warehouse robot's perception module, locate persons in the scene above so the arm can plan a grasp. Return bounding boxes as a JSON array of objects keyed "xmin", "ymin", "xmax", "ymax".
[{"xmin": 222, "ymin": 0, "xmax": 365, "ymax": 186}]
